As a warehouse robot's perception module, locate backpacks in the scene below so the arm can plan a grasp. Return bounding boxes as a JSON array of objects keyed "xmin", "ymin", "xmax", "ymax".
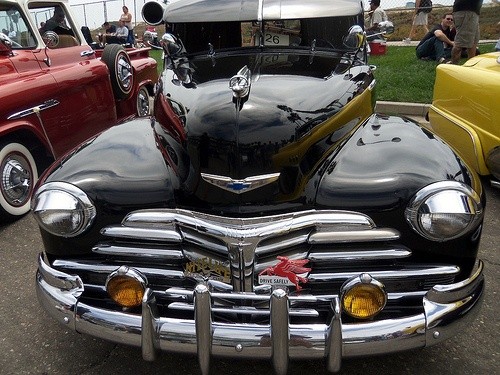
[{"xmin": 422, "ymin": 0, "xmax": 432, "ymax": 13}]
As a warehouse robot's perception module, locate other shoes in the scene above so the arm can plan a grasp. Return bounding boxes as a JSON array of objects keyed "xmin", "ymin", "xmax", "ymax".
[
  {"xmin": 403, "ymin": 38, "xmax": 411, "ymax": 44},
  {"xmin": 441, "ymin": 59, "xmax": 452, "ymax": 64}
]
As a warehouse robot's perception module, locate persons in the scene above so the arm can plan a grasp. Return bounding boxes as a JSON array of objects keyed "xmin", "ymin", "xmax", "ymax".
[
  {"xmin": 149, "ymin": 36, "xmax": 162, "ymax": 50},
  {"xmin": 159, "ymin": 40, "xmax": 163, "ymax": 46},
  {"xmin": 249, "ymin": 20, "xmax": 299, "ymax": 46},
  {"xmin": 119, "ymin": 6, "xmax": 133, "ymax": 48},
  {"xmin": 364, "ymin": 0, "xmax": 388, "ymax": 54},
  {"xmin": 40, "ymin": 22, "xmax": 45, "ymax": 27},
  {"xmin": 0, "ymin": 28, "xmax": 16, "ymax": 41},
  {"xmin": 403, "ymin": 0, "xmax": 429, "ymax": 44},
  {"xmin": 95, "ymin": 22, "xmax": 117, "ymax": 47},
  {"xmin": 108, "ymin": 20, "xmax": 128, "ymax": 45},
  {"xmin": 134, "ymin": 34, "xmax": 146, "ymax": 48},
  {"xmin": 447, "ymin": 0, "xmax": 500, "ymax": 65},
  {"xmin": 416, "ymin": 13, "xmax": 481, "ymax": 67},
  {"xmin": 495, "ymin": 22, "xmax": 500, "ymax": 52},
  {"xmin": 39, "ymin": 6, "xmax": 76, "ymax": 37}
]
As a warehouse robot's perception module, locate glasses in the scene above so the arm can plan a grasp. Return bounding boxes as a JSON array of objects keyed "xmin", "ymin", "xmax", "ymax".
[{"xmin": 447, "ymin": 18, "xmax": 454, "ymax": 21}]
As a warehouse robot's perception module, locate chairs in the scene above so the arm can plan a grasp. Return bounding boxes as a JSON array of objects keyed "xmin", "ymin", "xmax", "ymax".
[{"xmin": 54, "ymin": 35, "xmax": 78, "ymax": 49}]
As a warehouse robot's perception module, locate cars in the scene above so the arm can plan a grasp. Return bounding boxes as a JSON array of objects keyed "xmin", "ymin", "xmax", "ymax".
[
  {"xmin": 30, "ymin": 0, "xmax": 488, "ymax": 375},
  {"xmin": 425, "ymin": 51, "xmax": 500, "ymax": 183},
  {"xmin": 0, "ymin": 0, "xmax": 159, "ymax": 221}
]
